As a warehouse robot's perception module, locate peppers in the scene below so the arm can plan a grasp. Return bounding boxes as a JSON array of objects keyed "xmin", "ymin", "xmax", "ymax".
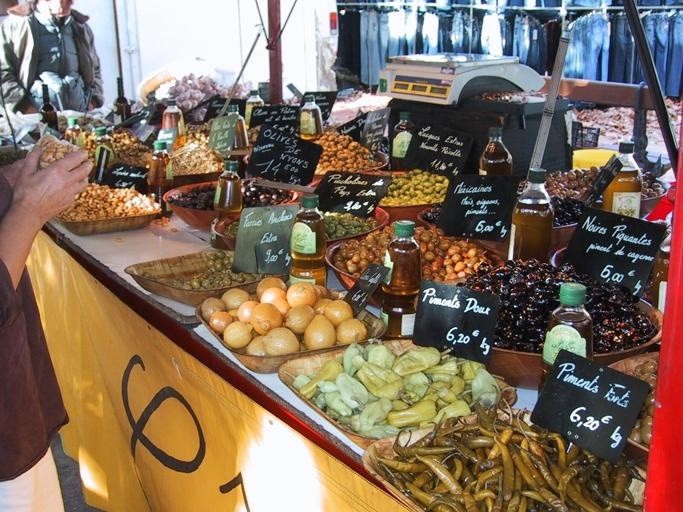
[
  {"xmin": 285, "ymin": 334, "xmax": 503, "ymax": 439},
  {"xmin": 373, "ymin": 391, "xmax": 646, "ymax": 511}
]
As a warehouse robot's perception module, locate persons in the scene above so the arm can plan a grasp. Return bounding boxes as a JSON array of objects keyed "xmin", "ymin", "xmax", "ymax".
[
  {"xmin": 0, "ymin": 0, "xmax": 105, "ymax": 115},
  {"xmin": 3, "ymin": 139, "xmax": 98, "ymax": 511}
]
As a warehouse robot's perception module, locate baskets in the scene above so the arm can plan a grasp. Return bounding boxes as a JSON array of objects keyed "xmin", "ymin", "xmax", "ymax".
[
  {"xmin": 123, "ymin": 249, "xmax": 290, "ymax": 308},
  {"xmin": 195, "ymin": 288, "xmax": 388, "ymax": 374},
  {"xmin": 276, "ymin": 339, "xmax": 517, "ymax": 451},
  {"xmin": 361, "ymin": 408, "xmax": 534, "ymax": 512},
  {"xmin": 52, "ymin": 188, "xmax": 163, "ymax": 236}
]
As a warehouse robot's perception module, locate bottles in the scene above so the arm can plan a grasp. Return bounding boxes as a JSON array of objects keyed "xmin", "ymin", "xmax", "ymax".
[
  {"xmin": 64, "ymin": 117, "xmax": 84, "ymax": 152},
  {"xmin": 300, "ymin": 95, "xmax": 323, "ymax": 141},
  {"xmin": 286, "ymin": 195, "xmax": 328, "ymax": 289},
  {"xmin": 538, "ymin": 281, "xmax": 594, "ymax": 401},
  {"xmin": 161, "ymin": 99, "xmax": 186, "ymax": 153},
  {"xmin": 225, "ymin": 104, "xmax": 251, "ymax": 179},
  {"xmin": 244, "ymin": 90, "xmax": 265, "ymax": 132},
  {"xmin": 380, "ymin": 219, "xmax": 424, "ymax": 340},
  {"xmin": 648, "ymin": 247, "xmax": 669, "ymax": 351},
  {"xmin": 94, "ymin": 126, "xmax": 115, "ymax": 179},
  {"xmin": 477, "ymin": 126, "xmax": 513, "ymax": 178},
  {"xmin": 507, "ymin": 167, "xmax": 554, "ymax": 262},
  {"xmin": 209, "ymin": 161, "xmax": 242, "ymax": 248},
  {"xmin": 113, "ymin": 78, "xmax": 130, "ymax": 128},
  {"xmin": 37, "ymin": 84, "xmax": 59, "ymax": 142},
  {"xmin": 390, "ymin": 111, "xmax": 417, "ymax": 172},
  {"xmin": 600, "ymin": 140, "xmax": 642, "ymax": 220},
  {"xmin": 146, "ymin": 141, "xmax": 173, "ymax": 219}
]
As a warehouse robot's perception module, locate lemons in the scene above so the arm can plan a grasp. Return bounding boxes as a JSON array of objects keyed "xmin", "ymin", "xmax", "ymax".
[{"xmin": 198, "ymin": 277, "xmax": 366, "ymax": 358}]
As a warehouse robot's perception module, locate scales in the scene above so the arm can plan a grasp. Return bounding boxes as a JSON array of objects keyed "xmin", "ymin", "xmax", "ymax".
[{"xmin": 375, "ymin": 52, "xmax": 547, "ymax": 108}]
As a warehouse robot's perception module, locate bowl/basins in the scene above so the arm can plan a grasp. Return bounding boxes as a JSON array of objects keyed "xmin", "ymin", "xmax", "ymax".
[
  {"xmin": 160, "ymin": 181, "xmax": 300, "ymax": 232},
  {"xmin": 325, "ymin": 228, "xmax": 503, "ymax": 309},
  {"xmin": 209, "ymin": 199, "xmax": 391, "ymax": 253},
  {"xmin": 601, "ymin": 352, "xmax": 658, "ymax": 466},
  {"xmin": 482, "ymin": 295, "xmax": 664, "ymax": 388},
  {"xmin": 144, "ymin": 171, "xmax": 229, "ymax": 204},
  {"xmin": 243, "ymin": 142, "xmax": 389, "ymax": 195},
  {"xmin": 592, "ymin": 179, "xmax": 669, "ymax": 220},
  {"xmin": 416, "ymin": 198, "xmax": 578, "ymax": 264},
  {"xmin": 354, "ymin": 170, "xmax": 442, "ymax": 226}
]
